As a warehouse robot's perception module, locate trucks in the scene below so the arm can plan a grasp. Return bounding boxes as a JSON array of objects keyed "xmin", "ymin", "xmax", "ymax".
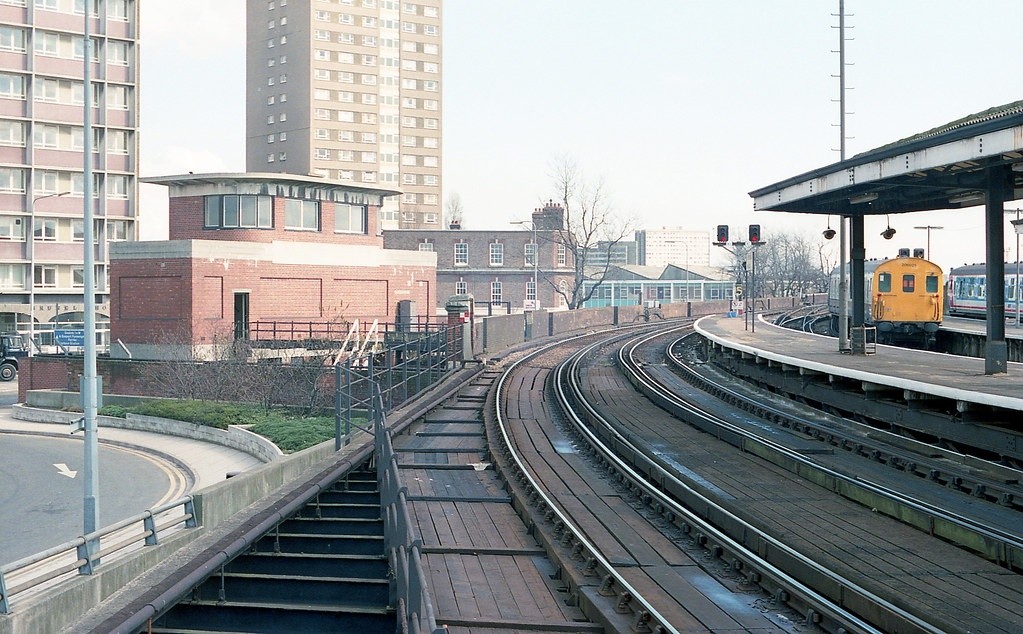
[{"xmin": 0, "ymin": 333, "xmax": 28, "ymax": 380}]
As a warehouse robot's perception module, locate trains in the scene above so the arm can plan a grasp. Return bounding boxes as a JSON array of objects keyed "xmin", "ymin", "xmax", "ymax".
[
  {"xmin": 947, "ymin": 263, "xmax": 1023, "ymax": 320},
  {"xmin": 827, "ymin": 248, "xmax": 945, "ymax": 351}
]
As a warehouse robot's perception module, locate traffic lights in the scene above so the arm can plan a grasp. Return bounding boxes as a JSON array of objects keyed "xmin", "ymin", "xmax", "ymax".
[
  {"xmin": 748, "ymin": 225, "xmax": 760, "ymax": 242},
  {"xmin": 717, "ymin": 224, "xmax": 728, "ymax": 242}
]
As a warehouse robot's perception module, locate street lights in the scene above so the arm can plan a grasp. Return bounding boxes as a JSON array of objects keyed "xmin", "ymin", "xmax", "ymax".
[
  {"xmin": 915, "ymin": 226, "xmax": 943, "ymax": 260},
  {"xmin": 1005, "ymin": 209, "xmax": 1023, "ymax": 327},
  {"xmin": 29, "ymin": 191, "xmax": 72, "ymax": 357},
  {"xmin": 664, "ymin": 240, "xmax": 689, "ymax": 318},
  {"xmin": 512, "ymin": 220, "xmax": 537, "ymax": 312}
]
